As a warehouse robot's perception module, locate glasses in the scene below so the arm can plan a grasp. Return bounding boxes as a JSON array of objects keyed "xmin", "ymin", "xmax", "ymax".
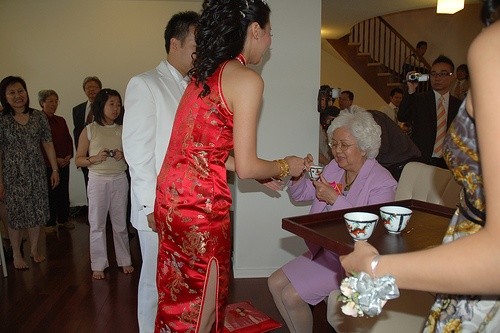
[
  {"xmin": 328, "ymin": 142, "xmax": 356, "ymax": 152},
  {"xmin": 431, "ymin": 70, "xmax": 452, "ymax": 79}
]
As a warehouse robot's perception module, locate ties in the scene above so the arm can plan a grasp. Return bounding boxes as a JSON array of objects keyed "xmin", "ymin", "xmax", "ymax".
[
  {"xmin": 85, "ymin": 104, "xmax": 94, "ymax": 124},
  {"xmin": 393, "ymin": 110, "xmax": 399, "ymax": 124},
  {"xmin": 180, "ymin": 80, "xmax": 189, "ymax": 91},
  {"xmin": 433, "ymin": 96, "xmax": 448, "ymax": 157}
]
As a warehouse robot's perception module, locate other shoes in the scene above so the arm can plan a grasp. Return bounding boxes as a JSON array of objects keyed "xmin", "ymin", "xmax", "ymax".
[
  {"xmin": 44, "ymin": 226, "xmax": 56, "ymax": 233},
  {"xmin": 57, "ymin": 222, "xmax": 75, "ymax": 228}
]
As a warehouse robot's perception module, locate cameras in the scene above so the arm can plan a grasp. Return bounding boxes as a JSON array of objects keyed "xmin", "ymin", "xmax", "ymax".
[
  {"xmin": 105, "ymin": 150, "xmax": 116, "ymax": 157},
  {"xmin": 408, "ymin": 73, "xmax": 430, "ymax": 82}
]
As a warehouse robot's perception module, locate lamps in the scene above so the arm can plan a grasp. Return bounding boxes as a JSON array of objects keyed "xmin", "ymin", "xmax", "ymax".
[{"xmin": 435, "ymin": 0, "xmax": 465, "ymax": 15}]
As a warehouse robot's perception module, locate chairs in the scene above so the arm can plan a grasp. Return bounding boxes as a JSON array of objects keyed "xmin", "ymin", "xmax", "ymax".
[{"xmin": 396, "ymin": 162, "xmax": 464, "ymax": 209}]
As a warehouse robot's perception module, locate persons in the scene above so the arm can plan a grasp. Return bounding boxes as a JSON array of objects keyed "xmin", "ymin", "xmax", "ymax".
[
  {"xmin": 388, "ymin": 87, "xmax": 403, "ymax": 112},
  {"xmin": 269, "ymin": 107, "xmax": 398, "ymax": 333},
  {"xmin": 398, "ymin": 55, "xmax": 462, "ymax": 171},
  {"xmin": 450, "ymin": 64, "xmax": 469, "ymax": 102},
  {"xmin": 76, "ymin": 89, "xmax": 134, "ymax": 279},
  {"xmin": 37, "ymin": 89, "xmax": 74, "ymax": 233},
  {"xmin": 72, "ymin": 77, "xmax": 131, "ymax": 230},
  {"xmin": 121, "ymin": 10, "xmax": 202, "ymax": 332},
  {"xmin": 153, "ymin": 0, "xmax": 305, "ymax": 333},
  {"xmin": 338, "ymin": 0, "xmax": 499, "ymax": 333},
  {"xmin": 319, "ymin": 85, "xmax": 353, "ymax": 169},
  {"xmin": 404, "ymin": 41, "xmax": 430, "ymax": 71},
  {"xmin": 0, "ymin": 76, "xmax": 59, "ymax": 269}
]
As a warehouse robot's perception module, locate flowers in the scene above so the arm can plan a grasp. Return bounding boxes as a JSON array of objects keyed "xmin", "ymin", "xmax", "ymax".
[{"xmin": 338, "ymin": 272, "xmax": 401, "ymax": 318}]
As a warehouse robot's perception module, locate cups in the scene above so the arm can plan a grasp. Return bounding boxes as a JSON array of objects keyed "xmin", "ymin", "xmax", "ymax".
[
  {"xmin": 344, "ymin": 212, "xmax": 379, "ymax": 242},
  {"xmin": 380, "ymin": 206, "xmax": 413, "ymax": 234},
  {"xmin": 308, "ymin": 165, "xmax": 324, "ymax": 181}
]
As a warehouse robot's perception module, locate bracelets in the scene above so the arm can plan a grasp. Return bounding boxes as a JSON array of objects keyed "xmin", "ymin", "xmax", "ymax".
[
  {"xmin": 53, "ymin": 169, "xmax": 58, "ymax": 172},
  {"xmin": 340, "ymin": 254, "xmax": 400, "ymax": 319},
  {"xmin": 343, "ymin": 170, "xmax": 357, "ymax": 191},
  {"xmin": 273, "ymin": 157, "xmax": 289, "ymax": 179}
]
